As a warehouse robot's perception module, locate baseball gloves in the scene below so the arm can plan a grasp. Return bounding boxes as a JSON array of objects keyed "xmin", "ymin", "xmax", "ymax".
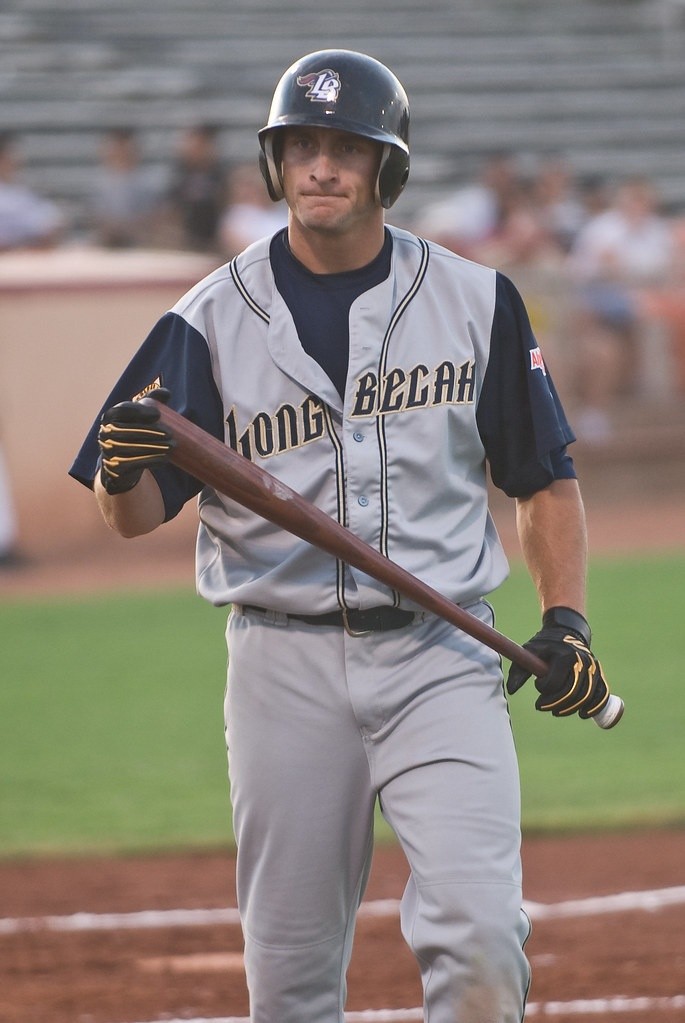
[{"xmin": 136, "ymin": 397, "xmax": 625, "ymax": 730}]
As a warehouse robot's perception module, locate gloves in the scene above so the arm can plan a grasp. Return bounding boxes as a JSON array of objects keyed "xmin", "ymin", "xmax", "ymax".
[
  {"xmin": 98, "ymin": 388, "xmax": 173, "ymax": 497},
  {"xmin": 507, "ymin": 606, "xmax": 610, "ymax": 720}
]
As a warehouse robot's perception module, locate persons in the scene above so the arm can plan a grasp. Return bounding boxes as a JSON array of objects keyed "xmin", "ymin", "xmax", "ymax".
[{"xmin": 67, "ymin": 51, "xmax": 611, "ymax": 1023}]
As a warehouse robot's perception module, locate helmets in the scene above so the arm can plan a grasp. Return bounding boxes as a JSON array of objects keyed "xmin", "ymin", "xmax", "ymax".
[{"xmin": 258, "ymin": 50, "xmax": 413, "ymax": 211}]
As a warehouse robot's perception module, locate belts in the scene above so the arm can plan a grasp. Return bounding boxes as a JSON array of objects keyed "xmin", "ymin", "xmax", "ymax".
[{"xmin": 246, "ymin": 605, "xmax": 415, "ymax": 636}]
